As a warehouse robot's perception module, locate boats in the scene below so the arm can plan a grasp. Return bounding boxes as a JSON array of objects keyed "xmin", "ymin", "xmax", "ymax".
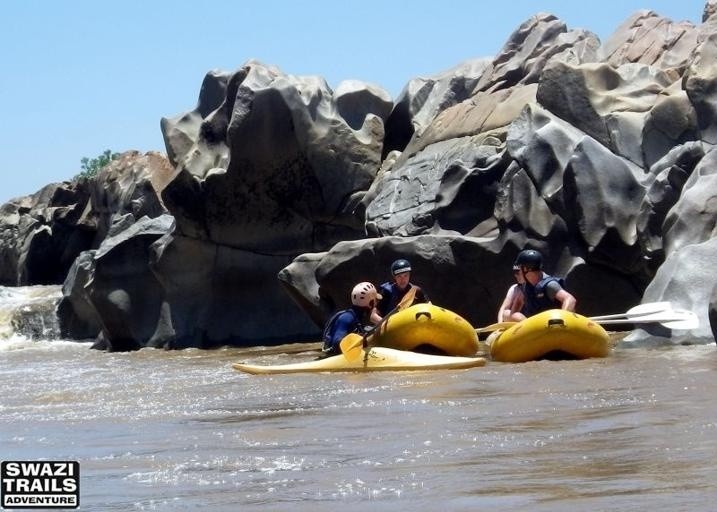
[
  {"xmin": 364, "ymin": 302, "xmax": 478, "ymax": 357},
  {"xmin": 490, "ymin": 308, "xmax": 611, "ymax": 362}
]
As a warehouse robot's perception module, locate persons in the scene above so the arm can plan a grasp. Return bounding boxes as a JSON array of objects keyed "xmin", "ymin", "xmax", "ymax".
[
  {"xmin": 318, "ymin": 281, "xmax": 381, "ymax": 360},
  {"xmin": 510, "ymin": 249, "xmax": 576, "ymax": 322},
  {"xmin": 495, "ymin": 260, "xmax": 524, "ymax": 325},
  {"xmin": 369, "ymin": 259, "xmax": 431, "ymax": 327}
]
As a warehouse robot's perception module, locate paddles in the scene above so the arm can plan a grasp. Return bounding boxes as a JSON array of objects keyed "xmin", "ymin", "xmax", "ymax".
[
  {"xmin": 339, "ymin": 287, "xmax": 416, "ymax": 363},
  {"xmin": 476, "ymin": 300, "xmax": 699, "ymax": 333}
]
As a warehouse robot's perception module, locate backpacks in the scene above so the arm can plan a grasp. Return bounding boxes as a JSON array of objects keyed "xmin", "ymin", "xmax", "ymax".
[{"xmin": 320, "ymin": 307, "xmax": 364, "ymax": 357}]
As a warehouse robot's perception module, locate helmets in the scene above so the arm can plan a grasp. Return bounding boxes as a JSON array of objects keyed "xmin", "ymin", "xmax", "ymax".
[
  {"xmin": 511, "ymin": 249, "xmax": 544, "ymax": 270},
  {"xmin": 350, "ymin": 281, "xmax": 383, "ymax": 310},
  {"xmin": 390, "ymin": 258, "xmax": 412, "ymax": 278}
]
